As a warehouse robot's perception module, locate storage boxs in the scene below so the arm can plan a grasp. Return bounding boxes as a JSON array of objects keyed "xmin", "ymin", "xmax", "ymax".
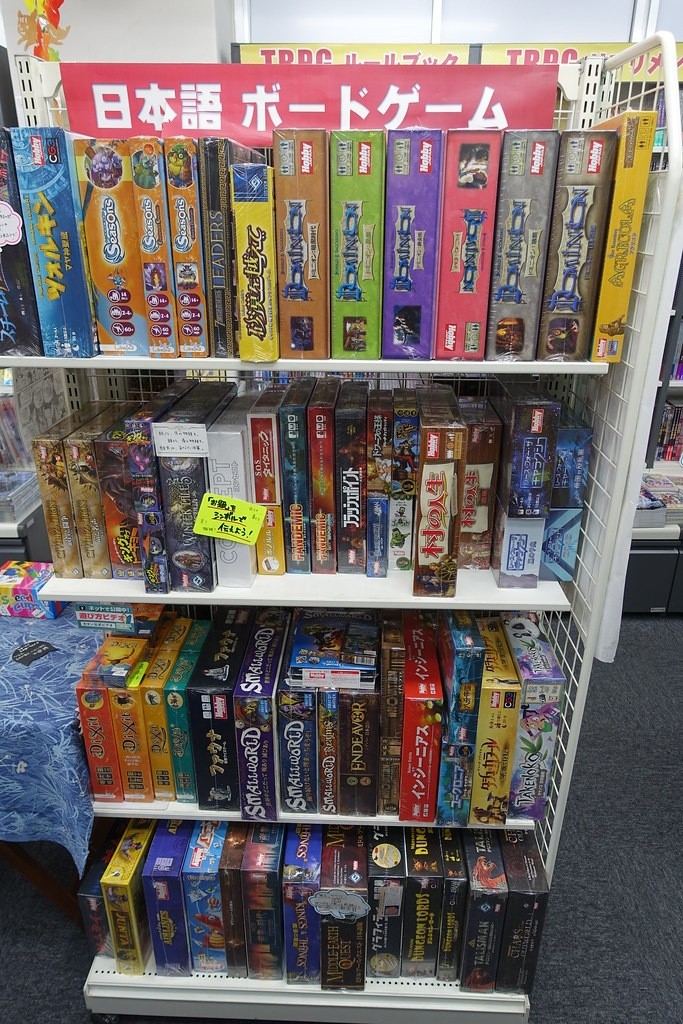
[
  {"xmin": 0, "ymin": 559, "xmax": 68, "ymax": 620},
  {"xmin": 76, "ymin": 821, "xmax": 551, "ymax": 998},
  {"xmin": 0, "ymin": 112, "xmax": 658, "ymax": 365},
  {"xmin": 75, "ymin": 604, "xmax": 568, "ymax": 818},
  {"xmin": 30, "ymin": 380, "xmax": 594, "ymax": 595}
]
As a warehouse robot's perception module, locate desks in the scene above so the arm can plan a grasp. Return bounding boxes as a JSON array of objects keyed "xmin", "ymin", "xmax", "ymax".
[{"xmin": 0, "ymin": 601, "xmax": 125, "ymax": 931}]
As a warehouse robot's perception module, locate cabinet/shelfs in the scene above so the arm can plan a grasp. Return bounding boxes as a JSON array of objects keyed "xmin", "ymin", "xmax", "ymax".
[
  {"xmin": 621, "ymin": 259, "xmax": 683, "ymax": 617},
  {"xmin": 0, "ymin": 30, "xmax": 683, "ymax": 1024}
]
